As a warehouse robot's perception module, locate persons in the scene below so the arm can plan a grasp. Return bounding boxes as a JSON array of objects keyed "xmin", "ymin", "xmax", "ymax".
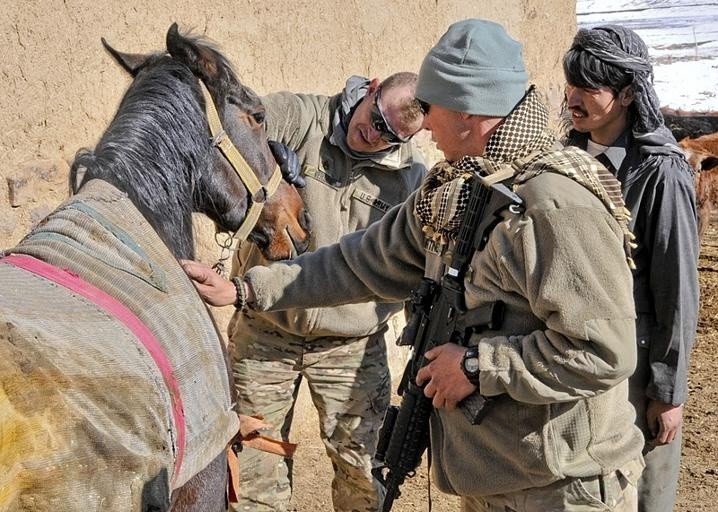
[
  {"xmin": 179, "ymin": 15, "xmax": 648, "ymax": 512},
  {"xmin": 226, "ymin": 70, "xmax": 432, "ymax": 512},
  {"xmin": 556, "ymin": 21, "xmax": 702, "ymax": 512}
]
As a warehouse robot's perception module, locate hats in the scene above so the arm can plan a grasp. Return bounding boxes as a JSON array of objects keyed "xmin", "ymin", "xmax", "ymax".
[{"xmin": 415, "ymin": 19, "xmax": 528, "ymax": 119}]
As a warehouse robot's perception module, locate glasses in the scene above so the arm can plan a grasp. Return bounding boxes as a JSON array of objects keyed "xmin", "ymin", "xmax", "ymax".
[
  {"xmin": 370, "ymin": 87, "xmax": 415, "ymax": 145},
  {"xmin": 414, "ymin": 98, "xmax": 429, "ymax": 116}
]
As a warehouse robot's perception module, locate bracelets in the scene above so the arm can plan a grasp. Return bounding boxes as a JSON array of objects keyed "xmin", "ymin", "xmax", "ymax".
[{"xmin": 233, "ymin": 275, "xmax": 248, "ymax": 316}]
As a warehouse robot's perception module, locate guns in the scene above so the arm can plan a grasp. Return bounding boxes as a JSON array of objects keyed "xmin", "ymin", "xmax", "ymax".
[{"xmin": 371, "ymin": 174, "xmax": 525, "ymax": 512}]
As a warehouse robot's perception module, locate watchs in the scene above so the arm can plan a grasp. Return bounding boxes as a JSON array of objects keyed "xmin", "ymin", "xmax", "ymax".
[{"xmin": 459, "ymin": 343, "xmax": 484, "ymax": 389}]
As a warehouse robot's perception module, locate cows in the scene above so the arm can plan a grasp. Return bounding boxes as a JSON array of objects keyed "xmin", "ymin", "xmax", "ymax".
[{"xmin": 678, "ymin": 131, "xmax": 718, "ymax": 240}]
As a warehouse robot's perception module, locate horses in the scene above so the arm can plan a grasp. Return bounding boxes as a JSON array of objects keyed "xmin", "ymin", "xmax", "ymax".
[{"xmin": 0, "ymin": 20, "xmax": 315, "ymax": 512}]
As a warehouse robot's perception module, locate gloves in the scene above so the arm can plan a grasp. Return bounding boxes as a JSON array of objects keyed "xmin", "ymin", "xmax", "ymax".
[{"xmin": 268, "ymin": 140, "xmax": 306, "ymax": 188}]
